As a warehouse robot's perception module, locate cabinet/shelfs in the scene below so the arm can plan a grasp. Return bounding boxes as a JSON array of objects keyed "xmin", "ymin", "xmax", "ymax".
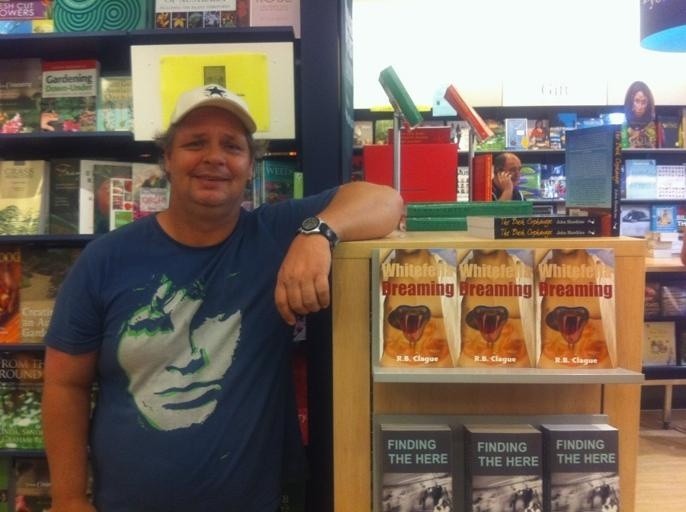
[
  {"xmin": 1, "ymin": 1, "xmax": 354, "ymax": 512},
  {"xmin": 330, "ymin": 238, "xmax": 652, "ymax": 512}
]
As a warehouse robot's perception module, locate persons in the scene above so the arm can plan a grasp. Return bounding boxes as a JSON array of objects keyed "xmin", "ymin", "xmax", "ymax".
[{"xmin": 39, "ymin": 84, "xmax": 405, "ymax": 512}]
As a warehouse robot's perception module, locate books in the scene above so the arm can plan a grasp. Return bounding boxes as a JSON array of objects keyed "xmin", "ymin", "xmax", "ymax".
[
  {"xmin": 0, "ymin": 248, "xmax": 93, "ymax": 512},
  {"xmin": 355, "ymin": 109, "xmax": 685, "ymax": 379},
  {"xmin": 377, "ymin": 420, "xmax": 621, "ymax": 512},
  {"xmin": 0, "ymin": 0, "xmax": 301, "ymax": 242}
]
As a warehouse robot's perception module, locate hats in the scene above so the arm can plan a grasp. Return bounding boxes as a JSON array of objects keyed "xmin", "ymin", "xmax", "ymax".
[{"xmin": 170, "ymin": 84, "xmax": 257, "ymax": 134}]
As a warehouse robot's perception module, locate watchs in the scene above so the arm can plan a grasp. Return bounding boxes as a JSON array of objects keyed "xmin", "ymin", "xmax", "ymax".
[{"xmin": 297, "ymin": 212, "xmax": 340, "ymax": 250}]
